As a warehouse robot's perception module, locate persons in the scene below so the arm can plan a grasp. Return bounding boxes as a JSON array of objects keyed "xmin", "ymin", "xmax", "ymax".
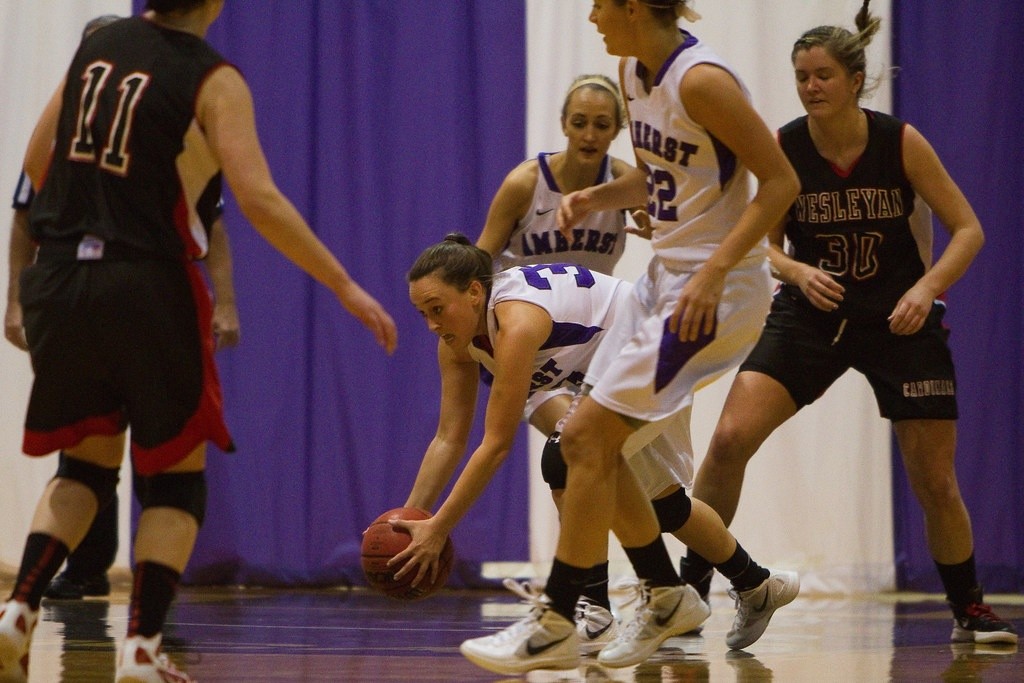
[
  {"xmin": 673, "ymin": 0, "xmax": 1020, "ymax": 644},
  {"xmin": 361, "ymin": 231, "xmax": 802, "ymax": 654},
  {"xmin": 0, "ymin": 0, "xmax": 399, "ymax": 683},
  {"xmin": 471, "ymin": 72, "xmax": 652, "ymax": 620},
  {"xmin": 459, "ymin": 0, "xmax": 800, "ymax": 672},
  {"xmin": 6, "ymin": 16, "xmax": 243, "ymax": 600}
]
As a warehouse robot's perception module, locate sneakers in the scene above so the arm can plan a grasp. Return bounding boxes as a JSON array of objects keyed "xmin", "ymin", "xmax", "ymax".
[
  {"xmin": 948, "ymin": 588, "xmax": 1020, "ymax": 646},
  {"xmin": 113, "ymin": 638, "xmax": 197, "ymax": 683},
  {"xmin": 571, "ymin": 595, "xmax": 620, "ymax": 655},
  {"xmin": 594, "ymin": 583, "xmax": 711, "ymax": 668},
  {"xmin": 0, "ymin": 599, "xmax": 37, "ymax": 683},
  {"xmin": 459, "ymin": 606, "xmax": 582, "ymax": 674},
  {"xmin": 42, "ymin": 570, "xmax": 111, "ymax": 601},
  {"xmin": 721, "ymin": 571, "xmax": 799, "ymax": 648},
  {"xmin": 676, "ymin": 557, "xmax": 714, "ymax": 607}
]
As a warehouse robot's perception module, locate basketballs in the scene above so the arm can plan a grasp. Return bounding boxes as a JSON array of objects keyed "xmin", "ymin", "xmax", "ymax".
[{"xmin": 360, "ymin": 507, "xmax": 454, "ymax": 602}]
